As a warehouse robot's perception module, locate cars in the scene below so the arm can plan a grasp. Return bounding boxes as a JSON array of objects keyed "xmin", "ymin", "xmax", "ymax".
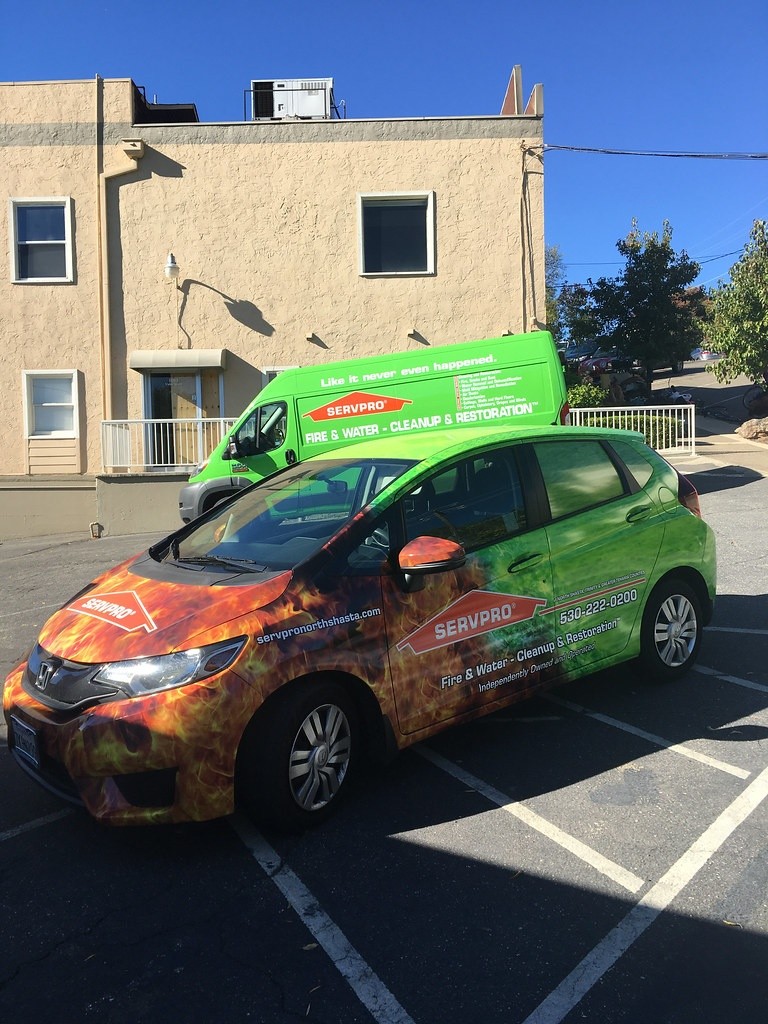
[{"xmin": 1, "ymin": 427, "xmax": 719, "ymax": 835}]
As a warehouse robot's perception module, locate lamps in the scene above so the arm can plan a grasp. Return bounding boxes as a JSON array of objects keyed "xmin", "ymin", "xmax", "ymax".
[
  {"xmin": 191, "ymin": 393, "xmax": 196, "ymax": 404},
  {"xmin": 406, "ymin": 328, "xmax": 415, "ymax": 335},
  {"xmin": 166, "ymin": 253, "xmax": 182, "ymax": 289},
  {"xmin": 306, "ymin": 331, "xmax": 314, "ymax": 339}
]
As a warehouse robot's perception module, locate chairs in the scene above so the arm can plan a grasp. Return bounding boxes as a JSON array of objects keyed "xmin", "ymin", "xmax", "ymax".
[
  {"xmin": 376, "ymin": 481, "xmax": 456, "ymax": 554},
  {"xmin": 468, "ymin": 460, "xmax": 521, "ymax": 520}
]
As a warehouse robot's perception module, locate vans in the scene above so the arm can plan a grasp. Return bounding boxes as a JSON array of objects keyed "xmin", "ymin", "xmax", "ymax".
[{"xmin": 178, "ymin": 329, "xmax": 571, "ymax": 528}]
